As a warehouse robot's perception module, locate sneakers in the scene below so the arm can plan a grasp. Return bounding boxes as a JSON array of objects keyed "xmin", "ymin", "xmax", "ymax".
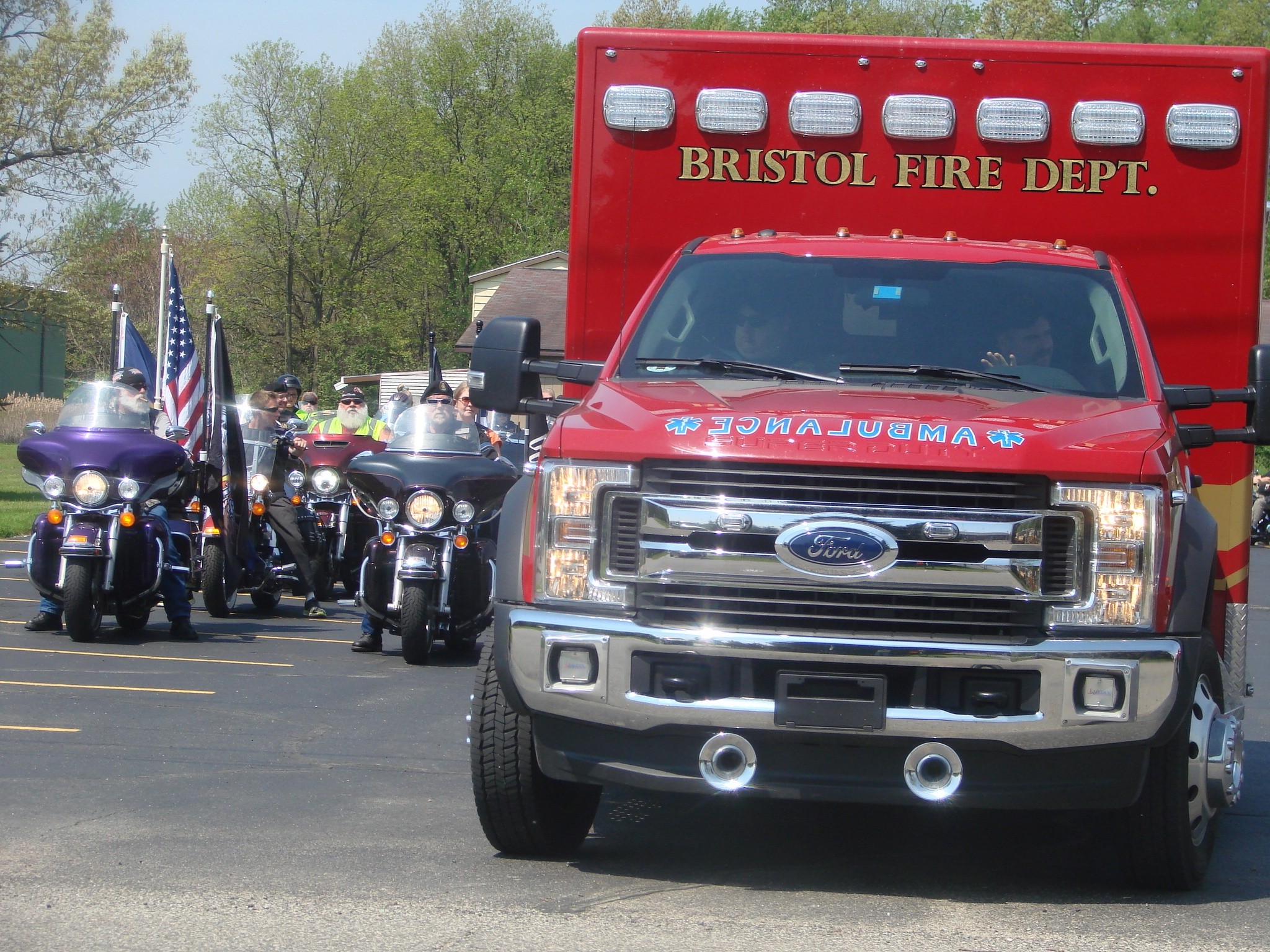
[
  {"xmin": 171, "ymin": 617, "xmax": 199, "ymax": 639},
  {"xmin": 24, "ymin": 611, "xmax": 63, "ymax": 631},
  {"xmin": 157, "ymin": 592, "xmax": 195, "ymax": 607},
  {"xmin": 351, "ymin": 631, "xmax": 383, "ymax": 651},
  {"xmin": 303, "ymin": 598, "xmax": 326, "ymax": 618}
]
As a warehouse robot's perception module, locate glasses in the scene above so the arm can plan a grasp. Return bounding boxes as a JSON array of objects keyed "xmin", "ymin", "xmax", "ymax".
[
  {"xmin": 131, "ymin": 385, "xmax": 147, "ymax": 391},
  {"xmin": 736, "ymin": 314, "xmax": 763, "ymax": 328},
  {"xmin": 339, "ymin": 399, "xmax": 363, "ymax": 405},
  {"xmin": 543, "ymin": 397, "xmax": 553, "ymax": 401},
  {"xmin": 303, "ymin": 401, "xmax": 318, "ymax": 405},
  {"xmin": 259, "ymin": 406, "xmax": 279, "ymax": 413},
  {"xmin": 426, "ymin": 398, "xmax": 452, "ymax": 406},
  {"xmin": 288, "ymin": 392, "xmax": 298, "ymax": 398},
  {"xmin": 458, "ymin": 397, "xmax": 471, "ymax": 403}
]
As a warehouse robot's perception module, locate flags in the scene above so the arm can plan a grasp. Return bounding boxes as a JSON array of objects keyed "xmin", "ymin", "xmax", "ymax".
[
  {"xmin": 160, "ymin": 253, "xmax": 208, "ymax": 464},
  {"xmin": 203, "ymin": 318, "xmax": 248, "ymax": 567},
  {"xmin": 119, "ymin": 314, "xmax": 162, "ymax": 403},
  {"xmin": 427, "ymin": 347, "xmax": 444, "ymax": 384}
]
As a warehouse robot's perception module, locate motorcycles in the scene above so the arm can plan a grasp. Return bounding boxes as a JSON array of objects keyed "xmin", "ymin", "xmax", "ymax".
[
  {"xmin": 16, "ymin": 381, "xmax": 199, "ymax": 644},
  {"xmin": 192, "ymin": 391, "xmax": 524, "ymax": 666}
]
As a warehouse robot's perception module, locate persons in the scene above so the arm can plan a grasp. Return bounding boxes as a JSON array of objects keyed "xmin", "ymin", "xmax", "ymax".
[
  {"xmin": 262, "ymin": 375, "xmax": 322, "ymax": 436},
  {"xmin": 972, "ymin": 286, "xmax": 1100, "ymax": 400},
  {"xmin": 291, "ymin": 385, "xmax": 395, "ymax": 600},
  {"xmin": 240, "ymin": 391, "xmax": 328, "ymax": 618},
  {"xmin": 351, "ymin": 381, "xmax": 490, "ymax": 652},
  {"xmin": 388, "ymin": 384, "xmax": 413, "ymax": 439},
  {"xmin": 452, "ymin": 381, "xmax": 502, "ymax": 462},
  {"xmin": 24, "ymin": 368, "xmax": 199, "ymax": 639},
  {"xmin": 678, "ymin": 288, "xmax": 818, "ymax": 376}
]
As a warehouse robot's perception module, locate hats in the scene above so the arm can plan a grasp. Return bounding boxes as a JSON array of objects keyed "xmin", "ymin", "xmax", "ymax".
[
  {"xmin": 112, "ymin": 366, "xmax": 146, "ymax": 386},
  {"xmin": 421, "ymin": 381, "xmax": 453, "ymax": 404},
  {"xmin": 340, "ymin": 385, "xmax": 365, "ymax": 399},
  {"xmin": 265, "ymin": 381, "xmax": 288, "ymax": 392}
]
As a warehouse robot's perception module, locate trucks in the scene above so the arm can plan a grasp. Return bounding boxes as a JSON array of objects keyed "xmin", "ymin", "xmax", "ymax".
[{"xmin": 468, "ymin": 26, "xmax": 1270, "ymax": 894}]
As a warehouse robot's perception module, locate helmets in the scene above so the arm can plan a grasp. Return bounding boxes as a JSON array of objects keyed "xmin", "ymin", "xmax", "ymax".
[{"xmin": 277, "ymin": 374, "xmax": 302, "ymax": 392}]
